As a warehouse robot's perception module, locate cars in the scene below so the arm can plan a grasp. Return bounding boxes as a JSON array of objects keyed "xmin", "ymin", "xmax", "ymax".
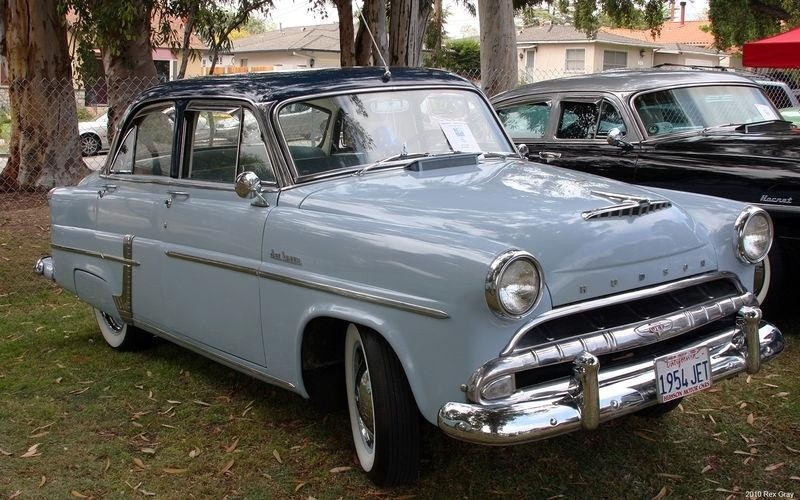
[
  {"xmin": 213, "ymin": 95, "xmax": 337, "ymax": 145},
  {"xmin": 75, "ymin": 104, "xmax": 211, "ymax": 156},
  {"xmin": 30, "ymin": 62, "xmax": 782, "ymax": 487},
  {"xmin": 485, "ymin": 69, "xmax": 800, "ymax": 313}
]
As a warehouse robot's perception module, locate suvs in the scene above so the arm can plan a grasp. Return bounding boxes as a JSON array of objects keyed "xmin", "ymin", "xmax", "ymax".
[{"xmin": 735, "ymin": 69, "xmax": 800, "ymax": 132}]
